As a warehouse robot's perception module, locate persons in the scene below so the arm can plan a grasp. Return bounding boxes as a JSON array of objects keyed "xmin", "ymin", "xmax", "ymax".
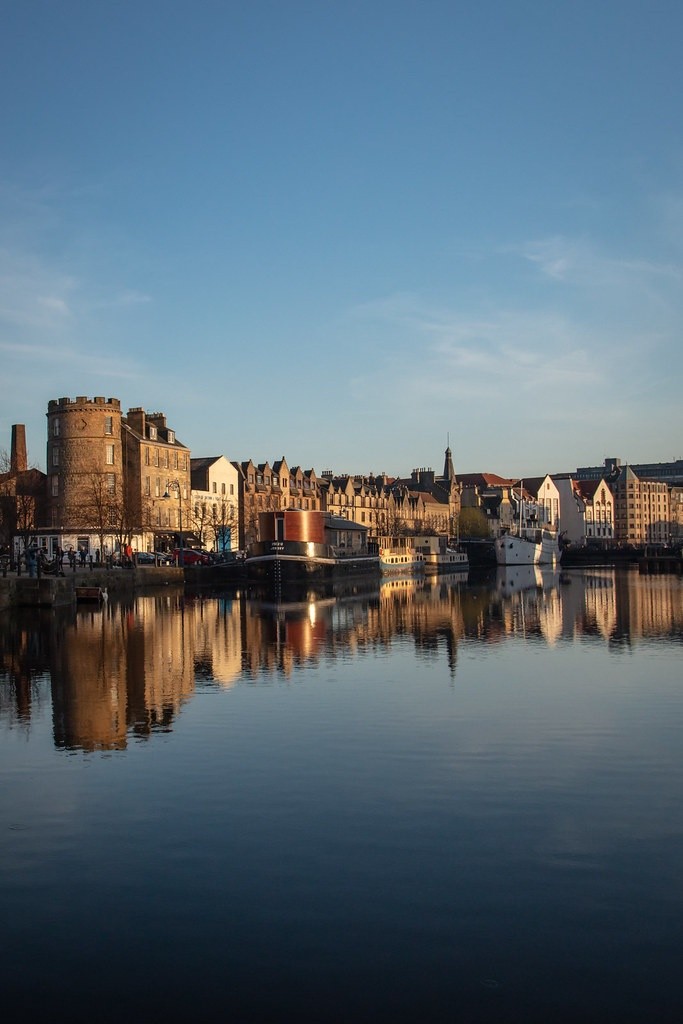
[
  {"xmin": 125, "ymin": 543, "xmax": 133, "ymax": 562},
  {"xmin": 95, "ymin": 549, "xmax": 100, "ymax": 562},
  {"xmin": 112, "ymin": 551, "xmax": 120, "ymax": 566},
  {"xmin": 29, "ymin": 551, "xmax": 37, "ymax": 577},
  {"xmin": 79, "ymin": 547, "xmax": 88, "ymax": 568},
  {"xmin": 67, "ymin": 547, "xmax": 77, "ymax": 568},
  {"xmin": 37, "ymin": 549, "xmax": 49, "ymax": 562},
  {"xmin": 55, "ymin": 545, "xmax": 65, "ymax": 572}
]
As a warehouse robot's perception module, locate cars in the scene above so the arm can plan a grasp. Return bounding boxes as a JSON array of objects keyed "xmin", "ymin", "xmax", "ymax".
[{"xmin": 133, "ymin": 548, "xmax": 229, "ymax": 567}]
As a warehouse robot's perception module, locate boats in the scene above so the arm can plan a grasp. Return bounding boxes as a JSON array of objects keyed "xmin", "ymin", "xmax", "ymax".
[{"xmin": 494, "ymin": 480, "xmax": 564, "ymax": 567}]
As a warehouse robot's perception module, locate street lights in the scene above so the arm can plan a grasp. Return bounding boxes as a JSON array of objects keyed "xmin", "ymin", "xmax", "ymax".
[{"xmin": 162, "ymin": 481, "xmax": 185, "ymax": 567}]
